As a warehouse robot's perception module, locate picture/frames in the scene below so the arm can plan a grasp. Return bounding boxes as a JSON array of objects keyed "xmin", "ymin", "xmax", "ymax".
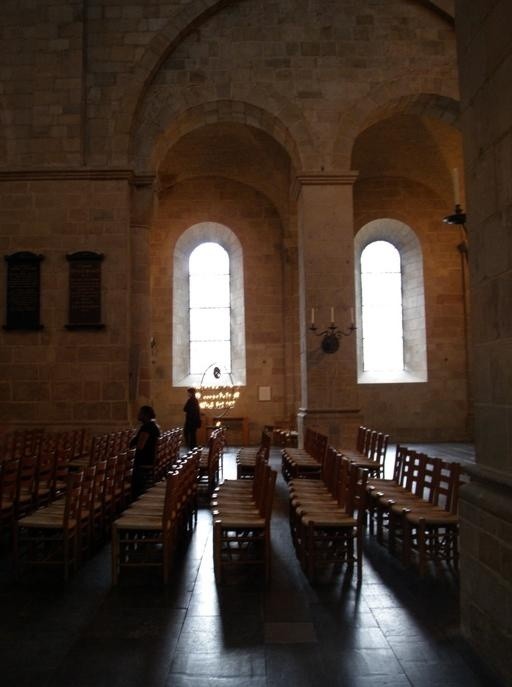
[{"xmin": 258, "ymin": 385, "xmax": 271, "ymax": 402}]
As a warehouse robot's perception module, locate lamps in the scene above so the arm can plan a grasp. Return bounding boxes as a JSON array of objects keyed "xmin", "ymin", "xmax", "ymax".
[{"xmin": 441, "ymin": 214, "xmax": 467, "ymax": 233}]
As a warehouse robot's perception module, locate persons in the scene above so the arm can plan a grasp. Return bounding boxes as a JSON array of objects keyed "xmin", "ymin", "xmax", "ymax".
[
  {"xmin": 127, "ymin": 405, "xmax": 161, "ymax": 496},
  {"xmin": 183, "ymin": 387, "xmax": 202, "ymax": 450}
]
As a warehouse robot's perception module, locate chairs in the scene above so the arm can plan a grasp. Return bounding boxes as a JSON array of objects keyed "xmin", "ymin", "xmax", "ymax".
[{"xmin": 0, "ymin": 426, "xmax": 461, "ymax": 591}]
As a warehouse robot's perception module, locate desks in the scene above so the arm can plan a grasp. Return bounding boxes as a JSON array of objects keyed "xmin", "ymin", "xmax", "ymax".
[{"xmin": 207, "ymin": 416, "xmax": 249, "ymax": 447}]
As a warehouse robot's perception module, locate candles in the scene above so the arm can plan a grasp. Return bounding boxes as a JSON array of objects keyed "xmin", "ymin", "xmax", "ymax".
[
  {"xmin": 331, "ymin": 306, "xmax": 334, "ymax": 323},
  {"xmin": 350, "ymin": 308, "xmax": 354, "ymax": 324},
  {"xmin": 195, "ymin": 392, "xmax": 240, "ymax": 410},
  {"xmin": 312, "ymin": 308, "xmax": 314, "ymax": 324}
]
